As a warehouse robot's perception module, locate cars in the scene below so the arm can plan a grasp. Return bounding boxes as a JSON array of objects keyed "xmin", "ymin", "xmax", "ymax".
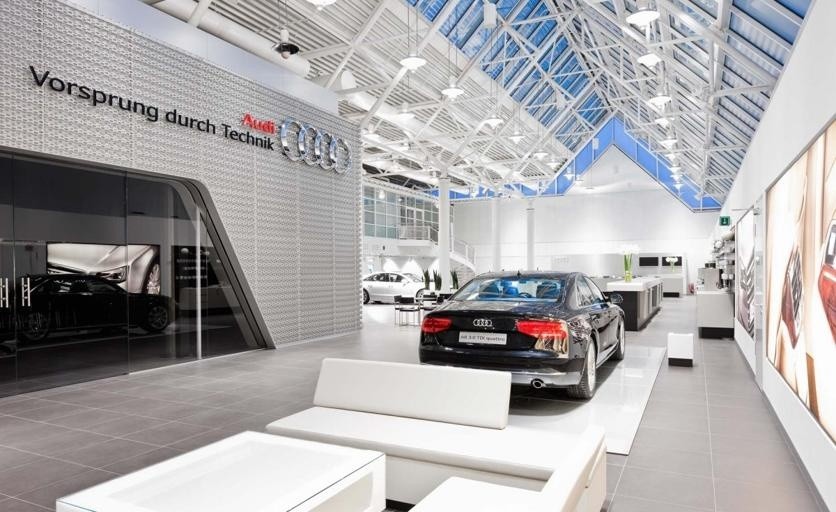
[
  {"xmin": 47, "ymin": 244, "xmax": 161, "ymax": 296},
  {"xmin": 417, "ymin": 265, "xmax": 628, "ymax": 406},
  {"xmin": 361, "ymin": 270, "xmax": 438, "ymax": 306},
  {"xmin": 0, "ymin": 273, "xmax": 179, "ymax": 343}
]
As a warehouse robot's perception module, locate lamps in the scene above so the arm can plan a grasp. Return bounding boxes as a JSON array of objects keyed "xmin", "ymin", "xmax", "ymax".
[
  {"xmin": 272, "ymin": 0, "xmax": 581, "ymax": 194},
  {"xmin": 627, "ymin": 0, "xmax": 684, "ymax": 195}
]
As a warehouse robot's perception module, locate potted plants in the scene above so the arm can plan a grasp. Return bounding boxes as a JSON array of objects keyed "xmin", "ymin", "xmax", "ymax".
[{"xmin": 624, "ymin": 254, "xmax": 633, "ymax": 284}]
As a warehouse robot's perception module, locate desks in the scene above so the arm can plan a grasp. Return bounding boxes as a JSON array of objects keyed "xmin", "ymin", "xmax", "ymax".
[{"xmin": 57, "ymin": 431, "xmax": 388, "ymax": 511}]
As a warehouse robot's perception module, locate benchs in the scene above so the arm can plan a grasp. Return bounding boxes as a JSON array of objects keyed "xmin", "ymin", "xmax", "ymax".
[{"xmin": 268, "ymin": 358, "xmax": 608, "ymax": 506}]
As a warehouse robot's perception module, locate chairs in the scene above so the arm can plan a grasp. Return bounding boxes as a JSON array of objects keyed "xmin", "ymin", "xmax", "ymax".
[{"xmin": 408, "ymin": 426, "xmax": 606, "ymax": 512}]
